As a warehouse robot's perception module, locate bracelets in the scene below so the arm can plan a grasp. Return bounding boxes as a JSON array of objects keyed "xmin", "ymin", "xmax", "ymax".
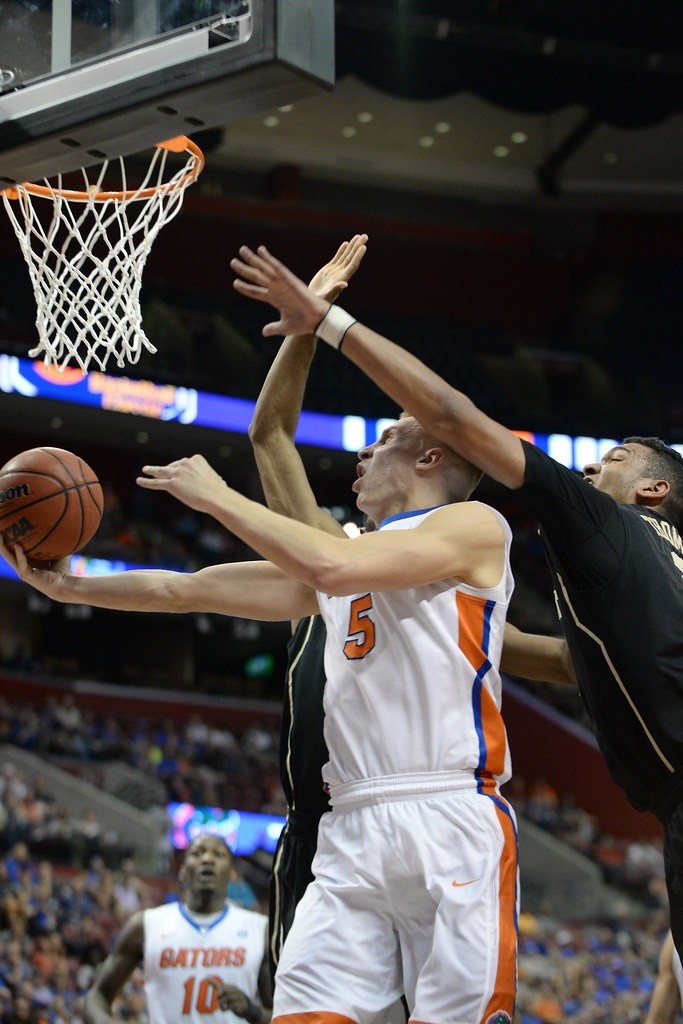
[{"xmin": 314, "ymin": 304, "xmax": 357, "ymax": 349}]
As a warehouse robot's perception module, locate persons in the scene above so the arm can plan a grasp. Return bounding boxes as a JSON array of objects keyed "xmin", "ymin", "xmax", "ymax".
[
  {"xmin": 231, "ymin": 247, "xmax": 683, "ymax": 969},
  {"xmin": 644, "ymin": 928, "xmax": 683, "ymax": 1024},
  {"xmin": 0, "ymin": 631, "xmax": 683, "ymax": 1024},
  {"xmin": 249, "ymin": 233, "xmax": 579, "ymax": 1024},
  {"xmin": 0, "ymin": 410, "xmax": 520, "ymax": 1024},
  {"xmin": 84, "ymin": 835, "xmax": 272, "ymax": 1024}
]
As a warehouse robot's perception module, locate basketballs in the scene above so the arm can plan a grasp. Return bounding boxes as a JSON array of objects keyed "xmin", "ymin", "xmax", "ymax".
[{"xmin": 0, "ymin": 444, "xmax": 106, "ymax": 563}]
